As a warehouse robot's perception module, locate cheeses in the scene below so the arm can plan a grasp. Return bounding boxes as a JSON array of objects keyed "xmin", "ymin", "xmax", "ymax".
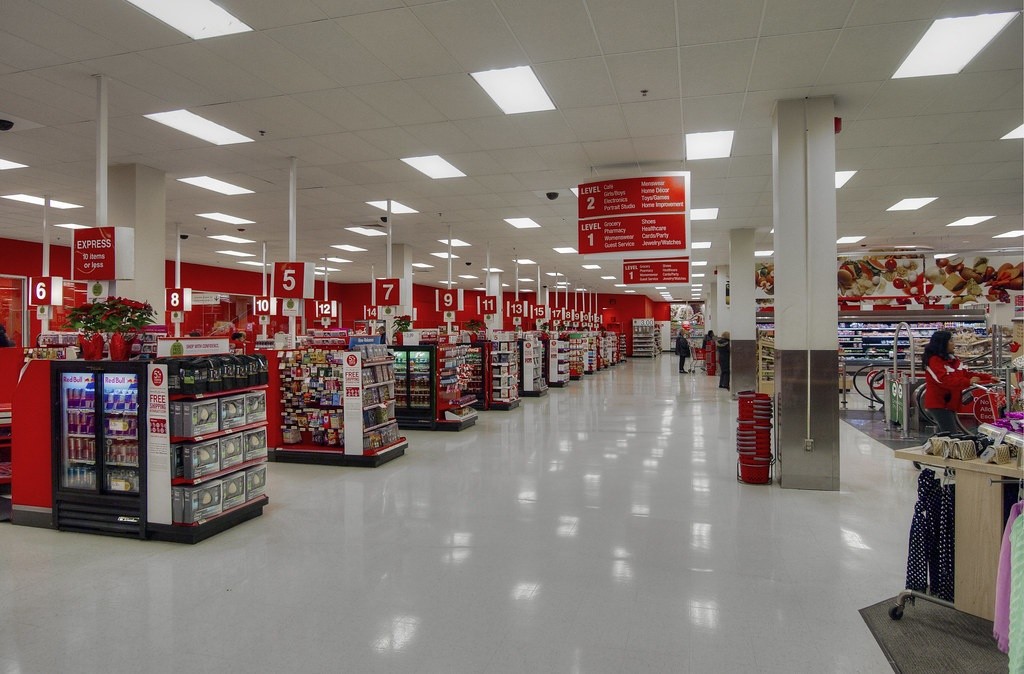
[{"xmin": 943, "ymin": 272, "xmax": 983, "ymax": 295}]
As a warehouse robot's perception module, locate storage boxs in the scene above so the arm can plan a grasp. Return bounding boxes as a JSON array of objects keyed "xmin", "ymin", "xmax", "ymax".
[
  {"xmin": 569, "ymin": 334, "xmax": 580, "ymax": 339},
  {"xmin": 349, "ymin": 336, "xmax": 381, "ymax": 351},
  {"xmin": 154, "ymin": 353, "xmax": 268, "ymax": 524}
]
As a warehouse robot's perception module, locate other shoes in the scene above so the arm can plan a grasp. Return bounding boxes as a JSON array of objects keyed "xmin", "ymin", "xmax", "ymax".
[
  {"xmin": 724, "ymin": 385, "xmax": 730, "ymax": 389},
  {"xmin": 719, "ymin": 385, "xmax": 723, "ymax": 388},
  {"xmin": 679, "ymin": 370, "xmax": 688, "ymax": 373}
]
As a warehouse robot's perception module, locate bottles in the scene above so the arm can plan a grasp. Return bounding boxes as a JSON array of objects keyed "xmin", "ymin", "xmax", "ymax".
[
  {"xmin": 944, "ymin": 322, "xmax": 960, "ymax": 328},
  {"xmin": 24, "ymin": 347, "xmax": 64, "ymax": 364},
  {"xmin": 550, "ymin": 333, "xmax": 558, "ymax": 339},
  {"xmin": 515, "ymin": 326, "xmax": 521, "ymax": 334}
]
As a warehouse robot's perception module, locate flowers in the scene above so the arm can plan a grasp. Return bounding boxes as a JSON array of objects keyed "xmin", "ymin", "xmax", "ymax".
[
  {"xmin": 539, "ymin": 322, "xmax": 550, "ymax": 331},
  {"xmin": 390, "ymin": 314, "xmax": 415, "ymax": 337},
  {"xmin": 555, "ymin": 322, "xmax": 567, "ymax": 331},
  {"xmin": 62, "ymin": 296, "xmax": 106, "ymax": 330},
  {"xmin": 463, "ymin": 319, "xmax": 488, "ymax": 334},
  {"xmin": 107, "ymin": 293, "xmax": 159, "ymax": 330}
]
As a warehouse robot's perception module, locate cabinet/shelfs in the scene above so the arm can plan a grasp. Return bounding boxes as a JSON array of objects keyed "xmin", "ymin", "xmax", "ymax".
[
  {"xmin": 168, "ymin": 353, "xmax": 269, "ymax": 527},
  {"xmin": 670, "ymin": 308, "xmax": 988, "ymax": 389},
  {"xmin": 268, "ymin": 317, "xmax": 662, "ymax": 544}
]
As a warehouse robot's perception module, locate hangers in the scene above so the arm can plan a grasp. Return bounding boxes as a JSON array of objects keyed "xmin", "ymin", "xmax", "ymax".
[{"xmin": 934, "ymin": 466, "xmax": 955, "ymax": 494}]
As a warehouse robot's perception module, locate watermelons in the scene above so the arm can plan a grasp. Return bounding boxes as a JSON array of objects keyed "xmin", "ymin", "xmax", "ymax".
[{"xmin": 866, "ymin": 259, "xmax": 887, "ymax": 271}]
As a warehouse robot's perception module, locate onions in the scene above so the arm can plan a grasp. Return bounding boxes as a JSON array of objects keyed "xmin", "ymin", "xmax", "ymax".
[{"xmin": 925, "ymin": 266, "xmax": 946, "ymax": 285}]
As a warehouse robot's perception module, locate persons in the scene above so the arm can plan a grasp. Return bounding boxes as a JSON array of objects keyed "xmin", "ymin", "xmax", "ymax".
[
  {"xmin": 232, "ymin": 333, "xmax": 245, "ymax": 342},
  {"xmin": 701, "ymin": 329, "xmax": 714, "ymax": 371},
  {"xmin": 716, "ymin": 332, "xmax": 730, "ymax": 389},
  {"xmin": 0, "ymin": 322, "xmax": 16, "ymax": 347},
  {"xmin": 675, "ymin": 328, "xmax": 690, "ymax": 373},
  {"xmin": 912, "ymin": 330, "xmax": 1000, "ymax": 480},
  {"xmin": 378, "ymin": 326, "xmax": 386, "ymax": 344}
]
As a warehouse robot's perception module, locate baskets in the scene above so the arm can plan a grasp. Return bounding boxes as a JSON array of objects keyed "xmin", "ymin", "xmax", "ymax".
[{"xmin": 736, "ymin": 390, "xmax": 773, "ymax": 485}]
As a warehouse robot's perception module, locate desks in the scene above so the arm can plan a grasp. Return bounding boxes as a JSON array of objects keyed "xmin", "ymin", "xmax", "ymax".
[{"xmin": 895, "ymin": 445, "xmax": 1024, "ymax": 623}]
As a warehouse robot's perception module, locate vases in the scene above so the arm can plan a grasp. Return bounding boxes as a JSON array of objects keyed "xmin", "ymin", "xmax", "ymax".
[
  {"xmin": 559, "ymin": 330, "xmax": 565, "ymax": 339},
  {"xmin": 109, "ymin": 328, "xmax": 132, "ymax": 360},
  {"xmin": 467, "ymin": 330, "xmax": 478, "ymax": 343},
  {"xmin": 542, "ymin": 329, "xmax": 548, "ymax": 339},
  {"xmin": 392, "ymin": 330, "xmax": 403, "ymax": 346},
  {"xmin": 78, "ymin": 331, "xmax": 103, "ymax": 360}
]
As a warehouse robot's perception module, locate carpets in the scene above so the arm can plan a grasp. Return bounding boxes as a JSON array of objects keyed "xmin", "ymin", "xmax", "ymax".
[{"xmin": 858, "ymin": 595, "xmax": 1009, "ymax": 674}]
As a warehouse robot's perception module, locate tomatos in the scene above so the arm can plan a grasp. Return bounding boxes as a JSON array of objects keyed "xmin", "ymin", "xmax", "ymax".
[
  {"xmin": 893, "ymin": 278, "xmax": 904, "ymax": 289},
  {"xmin": 885, "ymin": 259, "xmax": 898, "ymax": 270}
]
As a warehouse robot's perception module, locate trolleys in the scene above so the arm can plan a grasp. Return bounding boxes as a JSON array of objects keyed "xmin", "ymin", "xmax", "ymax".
[
  {"xmin": 688, "ymin": 346, "xmax": 706, "ymax": 374},
  {"xmin": 974, "ymin": 380, "xmax": 1018, "ymax": 426}
]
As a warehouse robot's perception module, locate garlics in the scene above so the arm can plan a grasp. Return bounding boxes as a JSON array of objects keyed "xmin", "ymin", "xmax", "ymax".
[
  {"xmin": 872, "ymin": 276, "xmax": 880, "ymax": 284},
  {"xmin": 910, "ymin": 286, "xmax": 919, "ymax": 294},
  {"xmin": 904, "ymin": 260, "xmax": 911, "ymax": 268},
  {"xmin": 907, "ymin": 270, "xmax": 918, "ymax": 282}
]
